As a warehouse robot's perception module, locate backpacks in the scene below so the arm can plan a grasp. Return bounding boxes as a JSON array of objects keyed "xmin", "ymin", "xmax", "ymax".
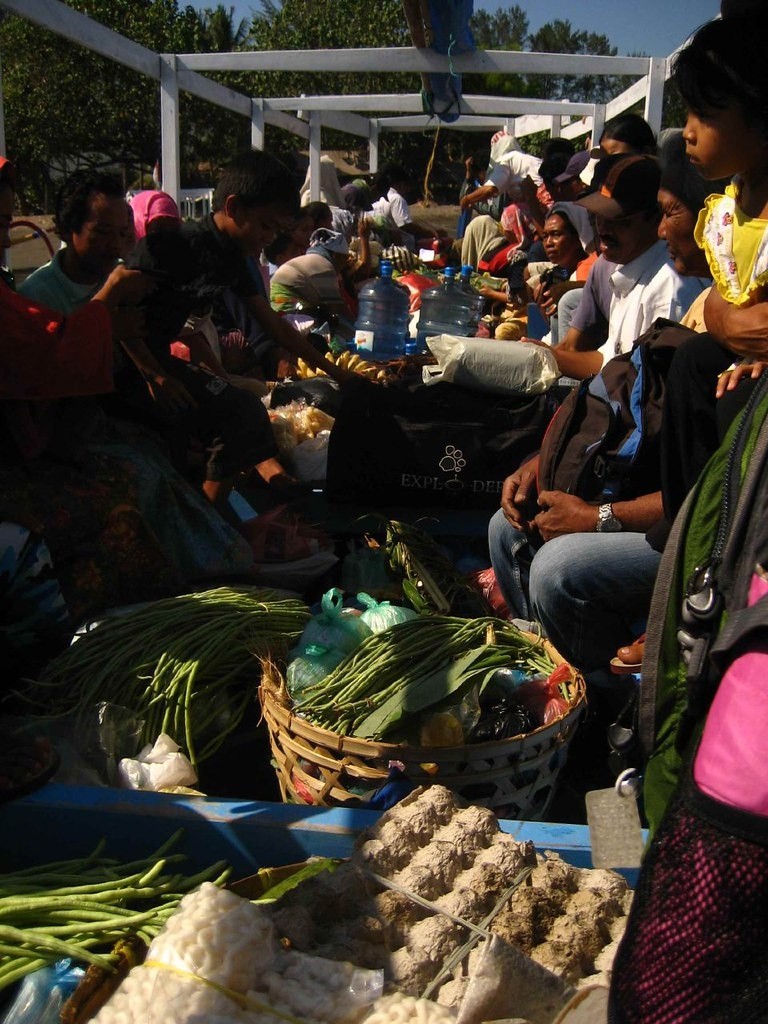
[{"xmin": 535, "ymin": 315, "xmax": 702, "ymax": 511}]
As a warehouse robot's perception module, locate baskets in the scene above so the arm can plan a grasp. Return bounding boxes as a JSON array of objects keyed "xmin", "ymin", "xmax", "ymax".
[
  {"xmin": 258, "ymin": 616, "xmax": 586, "ymax": 819},
  {"xmin": 58, "ymin": 858, "xmax": 336, "ymax": 1023}
]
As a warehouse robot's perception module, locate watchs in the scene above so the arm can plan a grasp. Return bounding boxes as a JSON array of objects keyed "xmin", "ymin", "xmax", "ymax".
[{"xmin": 596, "ymin": 503, "xmax": 624, "ymax": 531}]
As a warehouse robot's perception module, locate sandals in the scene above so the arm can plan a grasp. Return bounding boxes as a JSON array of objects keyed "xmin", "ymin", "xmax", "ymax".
[{"xmin": 610, "ymin": 629, "xmax": 647, "ymax": 675}]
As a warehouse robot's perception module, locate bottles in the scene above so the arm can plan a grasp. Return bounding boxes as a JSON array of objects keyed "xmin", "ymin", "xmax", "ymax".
[{"xmin": 344, "ymin": 259, "xmax": 485, "ymax": 362}]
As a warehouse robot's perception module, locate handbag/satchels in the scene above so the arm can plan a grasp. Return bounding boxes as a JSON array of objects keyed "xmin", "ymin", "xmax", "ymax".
[
  {"xmin": 606, "ymin": 770, "xmax": 768, "ymax": 1024},
  {"xmin": 236, "ymin": 501, "xmax": 330, "ymax": 562},
  {"xmin": 286, "ymin": 586, "xmax": 417, "ymax": 698},
  {"xmin": 323, "ymin": 382, "xmax": 579, "ymax": 508},
  {"xmin": 422, "ymin": 333, "xmax": 562, "ymax": 397}
]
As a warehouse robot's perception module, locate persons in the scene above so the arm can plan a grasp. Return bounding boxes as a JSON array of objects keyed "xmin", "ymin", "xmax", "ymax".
[{"xmin": 0, "ymin": 1, "xmax": 768, "ymax": 791}]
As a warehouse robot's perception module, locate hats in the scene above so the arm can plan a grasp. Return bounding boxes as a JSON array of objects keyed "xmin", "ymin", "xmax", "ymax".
[
  {"xmin": 575, "ymin": 155, "xmax": 660, "ymax": 222},
  {"xmin": 373, "ymin": 173, "xmax": 391, "ymax": 201},
  {"xmin": 554, "ymin": 151, "xmax": 589, "ymax": 184},
  {"xmin": 147, "ymin": 195, "xmax": 178, "ymax": 223},
  {"xmin": 341, "ymin": 179, "xmax": 373, "ymax": 211}
]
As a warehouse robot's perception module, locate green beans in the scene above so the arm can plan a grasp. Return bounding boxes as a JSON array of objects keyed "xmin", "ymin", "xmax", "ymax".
[
  {"xmin": 0, "ymin": 584, "xmax": 316, "ymax": 790},
  {"xmin": 290, "ymin": 615, "xmax": 569, "ymax": 739},
  {"xmin": 0, "ymin": 825, "xmax": 233, "ymax": 992}
]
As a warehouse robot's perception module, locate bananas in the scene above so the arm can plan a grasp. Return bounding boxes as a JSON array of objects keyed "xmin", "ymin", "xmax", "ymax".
[{"xmin": 296, "ymin": 349, "xmax": 385, "ymax": 383}]
